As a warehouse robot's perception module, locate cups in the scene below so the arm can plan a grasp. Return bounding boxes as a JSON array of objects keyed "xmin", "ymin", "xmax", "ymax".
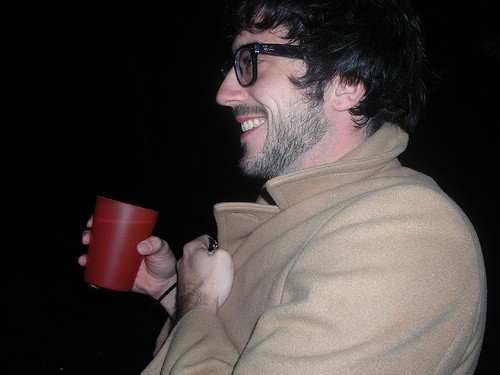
[{"xmin": 84, "ymin": 195, "xmax": 159, "ymax": 291}]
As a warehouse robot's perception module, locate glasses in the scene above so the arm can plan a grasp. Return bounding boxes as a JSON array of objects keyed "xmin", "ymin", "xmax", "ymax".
[{"xmin": 219, "ymin": 41, "xmax": 328, "ymax": 87}]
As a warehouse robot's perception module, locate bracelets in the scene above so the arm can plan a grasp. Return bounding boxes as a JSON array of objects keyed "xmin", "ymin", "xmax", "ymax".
[{"xmin": 157, "ymin": 278, "xmax": 177, "ymax": 305}]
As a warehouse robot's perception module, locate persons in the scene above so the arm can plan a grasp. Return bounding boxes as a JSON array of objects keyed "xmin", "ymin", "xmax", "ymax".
[{"xmin": 77, "ymin": 2, "xmax": 487, "ymax": 375}]
